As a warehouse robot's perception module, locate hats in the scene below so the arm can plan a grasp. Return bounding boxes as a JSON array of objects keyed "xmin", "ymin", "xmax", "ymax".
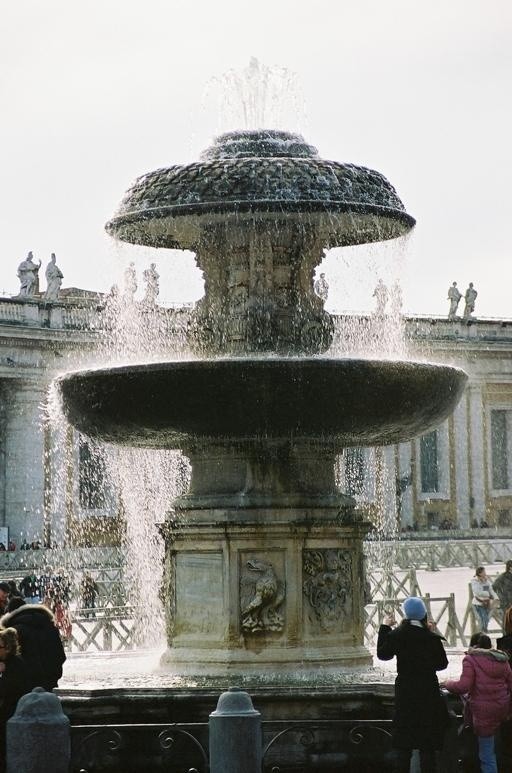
[{"xmin": 404, "ymin": 596, "xmax": 427, "ymax": 619}]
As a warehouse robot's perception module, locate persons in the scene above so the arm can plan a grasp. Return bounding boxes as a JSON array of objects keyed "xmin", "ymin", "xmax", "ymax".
[
  {"xmin": 144, "ymin": 263, "xmax": 160, "ymax": 308},
  {"xmin": 441, "ymin": 559, "xmax": 510, "ymax": 772},
  {"xmin": 0, "ymin": 536, "xmax": 99, "ymax": 772},
  {"xmin": 392, "ymin": 278, "xmax": 404, "ymax": 313},
  {"xmin": 46, "ymin": 252, "xmax": 66, "ymax": 300},
  {"xmin": 446, "ymin": 282, "xmax": 463, "ymax": 316},
  {"xmin": 316, "ymin": 271, "xmax": 329, "ymax": 310},
  {"xmin": 371, "ymin": 277, "xmax": 390, "ymax": 314},
  {"xmin": 464, "ymin": 281, "xmax": 478, "ymax": 318},
  {"xmin": 376, "ymin": 597, "xmax": 449, "ymax": 771},
  {"xmin": 16, "ymin": 250, "xmax": 43, "ymax": 298},
  {"xmin": 122, "ymin": 260, "xmax": 138, "ymax": 303}
]
